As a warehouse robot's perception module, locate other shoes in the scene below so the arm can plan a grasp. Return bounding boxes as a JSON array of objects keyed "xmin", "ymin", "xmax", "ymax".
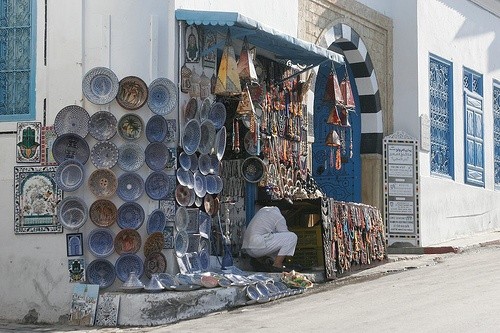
[
  {"xmin": 270, "ymin": 265, "xmax": 284, "ymax": 273},
  {"xmin": 250, "ymin": 260, "xmax": 267, "ymax": 271}
]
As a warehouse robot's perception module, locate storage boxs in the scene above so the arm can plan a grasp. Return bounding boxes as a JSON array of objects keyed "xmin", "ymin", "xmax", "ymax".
[
  {"xmin": 288, "ymin": 225, "xmax": 322, "ymax": 248},
  {"xmin": 289, "ymin": 247, "xmax": 325, "ymax": 271}
]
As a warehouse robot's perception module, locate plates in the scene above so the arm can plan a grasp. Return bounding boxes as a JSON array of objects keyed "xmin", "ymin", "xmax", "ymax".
[
  {"xmin": 146, "ymin": 209, "xmax": 166, "ymax": 235},
  {"xmin": 145, "ymin": 142, "xmax": 169, "ymax": 172},
  {"xmin": 147, "ymin": 76, "xmax": 177, "ymax": 115},
  {"xmin": 118, "ymin": 113, "xmax": 145, "ymax": 142},
  {"xmin": 90, "ymin": 199, "xmax": 118, "ymax": 227},
  {"xmin": 88, "ymin": 111, "xmax": 118, "ymax": 141},
  {"xmin": 86, "ymin": 227, "xmax": 116, "ymax": 258},
  {"xmin": 57, "ymin": 196, "xmax": 89, "ymax": 230},
  {"xmin": 113, "ymin": 253, "xmax": 144, "ymax": 280},
  {"xmin": 51, "ymin": 133, "xmax": 90, "ymax": 166},
  {"xmin": 87, "ymin": 168, "xmax": 118, "ymax": 198},
  {"xmin": 160, "ymin": 59, "xmax": 306, "ymax": 303},
  {"xmin": 115, "ymin": 229, "xmax": 141, "ymax": 256},
  {"xmin": 117, "ymin": 202, "xmax": 145, "ymax": 230},
  {"xmin": 116, "ymin": 172, "xmax": 144, "ymax": 202},
  {"xmin": 82, "ymin": 66, "xmax": 119, "ymax": 104},
  {"xmin": 117, "ymin": 143, "xmax": 145, "ymax": 172},
  {"xmin": 55, "ymin": 159, "xmax": 87, "ymax": 192},
  {"xmin": 117, "ymin": 75, "xmax": 148, "ymax": 110},
  {"xmin": 54, "ymin": 105, "xmax": 91, "ymax": 141},
  {"xmin": 145, "ymin": 253, "xmax": 166, "ymax": 279},
  {"xmin": 146, "ymin": 115, "xmax": 167, "ymax": 144},
  {"xmin": 143, "ymin": 233, "xmax": 164, "ymax": 257},
  {"xmin": 145, "ymin": 173, "xmax": 169, "ymax": 200},
  {"xmin": 91, "ymin": 141, "xmax": 119, "ymax": 168},
  {"xmin": 86, "ymin": 258, "xmax": 116, "ymax": 289}
]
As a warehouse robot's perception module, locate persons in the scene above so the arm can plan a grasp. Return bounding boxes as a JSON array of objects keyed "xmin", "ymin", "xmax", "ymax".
[{"xmin": 241, "ymin": 201, "xmax": 298, "ymax": 273}]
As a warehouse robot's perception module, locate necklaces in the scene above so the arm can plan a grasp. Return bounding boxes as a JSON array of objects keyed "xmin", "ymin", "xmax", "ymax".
[
  {"xmin": 321, "ymin": 199, "xmax": 385, "ymax": 278},
  {"xmin": 262, "ymin": 57, "xmax": 324, "ymax": 201}
]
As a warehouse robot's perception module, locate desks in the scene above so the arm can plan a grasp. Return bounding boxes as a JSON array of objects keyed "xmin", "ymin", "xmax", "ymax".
[{"xmin": 257, "ymin": 198, "xmax": 386, "ymax": 277}]
